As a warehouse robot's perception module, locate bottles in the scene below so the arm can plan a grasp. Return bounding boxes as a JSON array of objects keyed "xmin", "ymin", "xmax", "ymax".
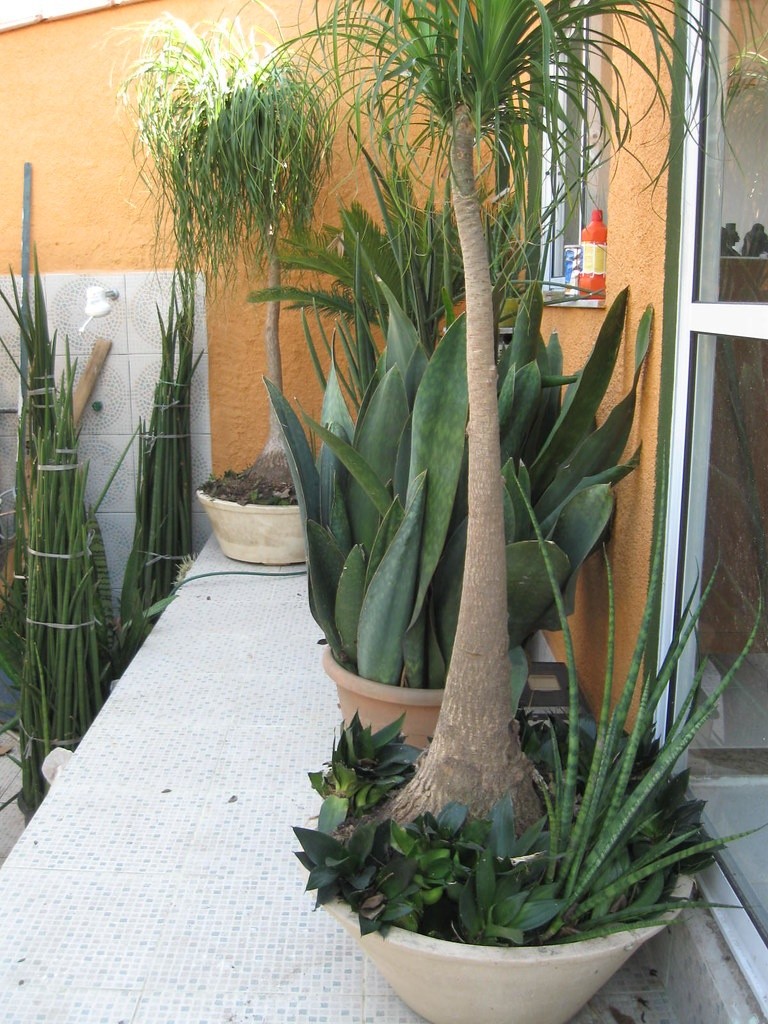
[{"xmin": 579, "ymin": 210, "xmax": 607, "ymax": 298}]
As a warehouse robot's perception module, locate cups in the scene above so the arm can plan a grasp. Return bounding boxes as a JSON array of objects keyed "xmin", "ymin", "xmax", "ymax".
[{"xmin": 564, "ymin": 245, "xmax": 582, "ymax": 296}]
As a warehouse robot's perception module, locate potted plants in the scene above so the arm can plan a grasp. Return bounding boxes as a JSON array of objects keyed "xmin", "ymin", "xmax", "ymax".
[{"xmin": 109, "ymin": 1, "xmax": 768, "ymax": 1024}]
